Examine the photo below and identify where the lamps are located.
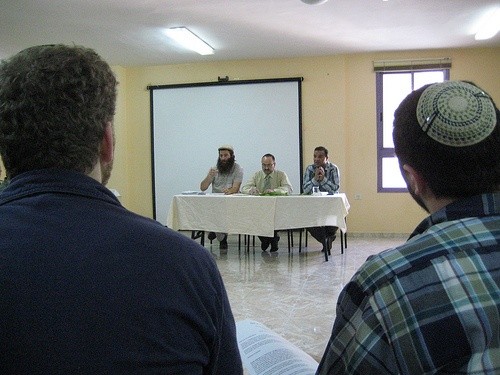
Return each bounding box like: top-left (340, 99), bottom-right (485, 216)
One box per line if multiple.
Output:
top-left (168, 24), bottom-right (215, 56)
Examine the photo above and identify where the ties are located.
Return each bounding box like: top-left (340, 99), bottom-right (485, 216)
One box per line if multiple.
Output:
top-left (264, 176), bottom-right (271, 189)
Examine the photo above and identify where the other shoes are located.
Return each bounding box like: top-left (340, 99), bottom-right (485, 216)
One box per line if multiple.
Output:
top-left (208, 232), bottom-right (217, 240)
top-left (261, 234), bottom-right (281, 252)
top-left (220, 235), bottom-right (228, 249)
top-left (321, 235), bottom-right (336, 252)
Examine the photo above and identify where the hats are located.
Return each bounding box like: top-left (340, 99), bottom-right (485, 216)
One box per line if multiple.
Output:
top-left (218, 144), bottom-right (234, 152)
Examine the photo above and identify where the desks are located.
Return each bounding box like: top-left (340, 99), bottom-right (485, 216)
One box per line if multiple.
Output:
top-left (165, 192), bottom-right (352, 258)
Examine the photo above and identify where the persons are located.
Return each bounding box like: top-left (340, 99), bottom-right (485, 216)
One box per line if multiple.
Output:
top-left (0, 42), bottom-right (244, 375)
top-left (302, 146), bottom-right (340, 251)
top-left (240, 153), bottom-right (292, 253)
top-left (317, 80), bottom-right (499, 375)
top-left (200, 146), bottom-right (244, 251)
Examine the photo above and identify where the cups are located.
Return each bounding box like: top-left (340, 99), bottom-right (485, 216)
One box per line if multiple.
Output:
top-left (251, 187), bottom-right (257, 196)
top-left (212, 166), bottom-right (218, 177)
top-left (313, 186), bottom-right (320, 196)
